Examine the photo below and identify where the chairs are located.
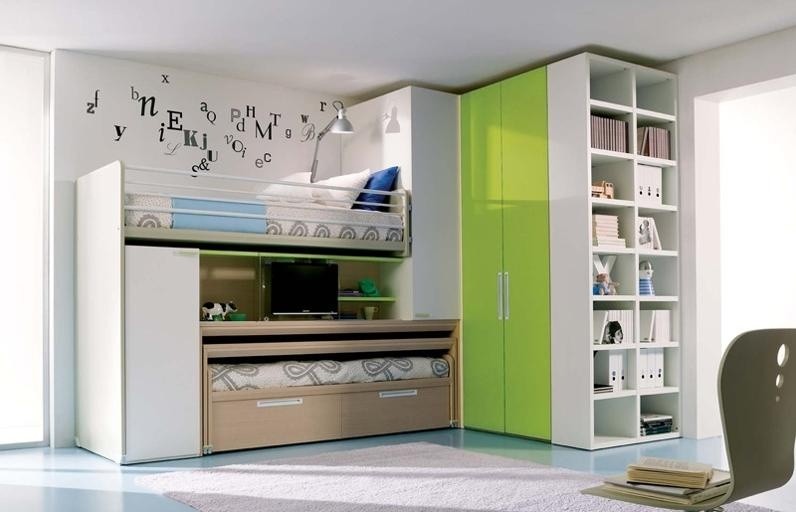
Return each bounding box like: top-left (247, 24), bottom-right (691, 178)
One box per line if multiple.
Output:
top-left (579, 328), bottom-right (795, 512)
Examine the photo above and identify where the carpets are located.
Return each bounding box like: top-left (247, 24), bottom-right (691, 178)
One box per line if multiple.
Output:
top-left (136, 440), bottom-right (777, 512)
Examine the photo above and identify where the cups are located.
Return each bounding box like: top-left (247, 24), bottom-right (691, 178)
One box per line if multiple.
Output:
top-left (364, 306), bottom-right (378, 320)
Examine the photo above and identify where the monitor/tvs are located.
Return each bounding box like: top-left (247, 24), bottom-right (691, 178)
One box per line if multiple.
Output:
top-left (271, 261), bottom-right (338, 319)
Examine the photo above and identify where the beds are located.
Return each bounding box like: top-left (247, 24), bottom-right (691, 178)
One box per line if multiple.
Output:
top-left (122, 159), bottom-right (411, 253)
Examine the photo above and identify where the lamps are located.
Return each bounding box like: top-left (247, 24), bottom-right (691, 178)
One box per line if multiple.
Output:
top-left (311, 108), bottom-right (354, 183)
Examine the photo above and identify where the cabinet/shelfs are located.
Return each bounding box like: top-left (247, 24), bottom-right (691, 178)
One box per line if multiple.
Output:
top-left (124, 245), bottom-right (460, 466)
top-left (460, 51), bottom-right (683, 450)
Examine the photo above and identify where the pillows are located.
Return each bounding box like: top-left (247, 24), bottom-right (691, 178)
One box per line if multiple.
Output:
top-left (254, 167), bottom-right (398, 211)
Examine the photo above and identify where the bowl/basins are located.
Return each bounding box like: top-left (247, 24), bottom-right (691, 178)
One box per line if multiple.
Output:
top-left (229, 313), bottom-right (248, 320)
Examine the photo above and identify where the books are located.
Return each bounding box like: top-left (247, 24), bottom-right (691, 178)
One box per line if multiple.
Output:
top-left (601, 484), bottom-right (732, 505)
top-left (601, 466), bottom-right (731, 495)
top-left (624, 455), bottom-right (713, 490)
top-left (589, 111), bottom-right (676, 439)
top-left (339, 289), bottom-right (361, 296)
